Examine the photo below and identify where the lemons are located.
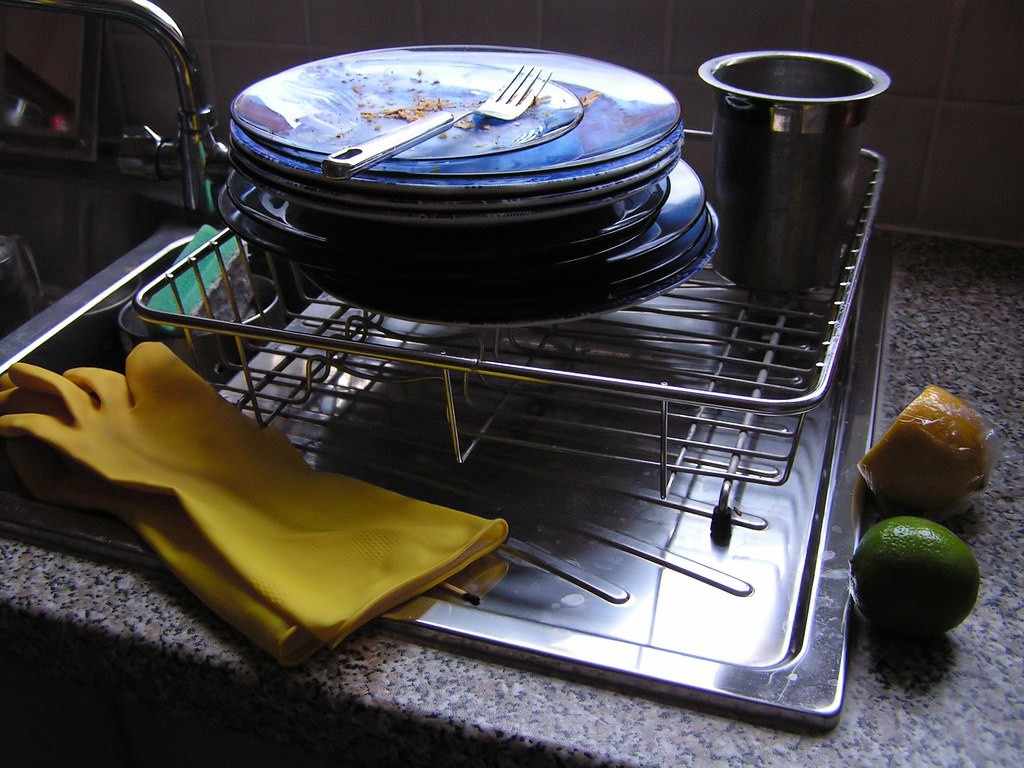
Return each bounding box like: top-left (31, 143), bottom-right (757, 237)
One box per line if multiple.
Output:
top-left (856, 385), bottom-right (993, 512)
top-left (849, 517), bottom-right (980, 634)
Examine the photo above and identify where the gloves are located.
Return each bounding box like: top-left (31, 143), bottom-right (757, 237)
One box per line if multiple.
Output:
top-left (0, 340), bottom-right (508, 667)
